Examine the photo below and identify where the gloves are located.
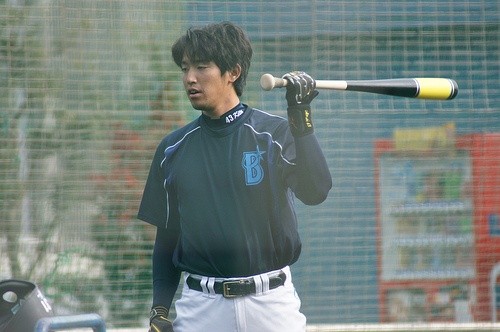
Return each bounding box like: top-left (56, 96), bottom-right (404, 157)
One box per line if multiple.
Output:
top-left (149, 306), bottom-right (173, 332)
top-left (282, 70), bottom-right (320, 139)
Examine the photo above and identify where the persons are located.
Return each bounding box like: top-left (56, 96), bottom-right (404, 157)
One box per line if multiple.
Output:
top-left (136, 20), bottom-right (333, 332)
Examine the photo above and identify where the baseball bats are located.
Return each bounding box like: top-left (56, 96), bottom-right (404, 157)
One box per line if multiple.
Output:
top-left (260, 73), bottom-right (459, 101)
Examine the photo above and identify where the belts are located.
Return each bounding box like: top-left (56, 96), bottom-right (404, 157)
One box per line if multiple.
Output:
top-left (185, 274), bottom-right (287, 298)
